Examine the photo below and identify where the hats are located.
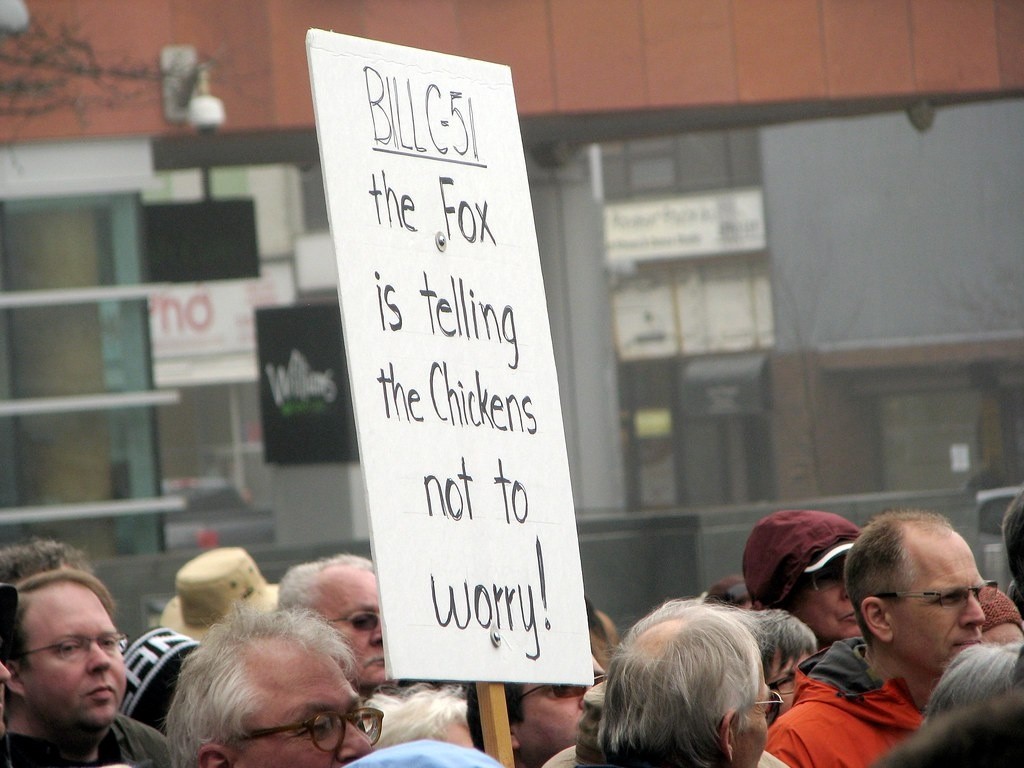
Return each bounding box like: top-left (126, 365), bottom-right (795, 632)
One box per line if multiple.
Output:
top-left (159, 544), bottom-right (282, 642)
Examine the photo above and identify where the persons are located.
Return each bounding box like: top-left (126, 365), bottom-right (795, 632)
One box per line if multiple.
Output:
top-left (0, 489), bottom-right (1024, 768)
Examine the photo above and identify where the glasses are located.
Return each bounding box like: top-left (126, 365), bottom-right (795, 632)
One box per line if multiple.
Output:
top-left (508, 672), bottom-right (609, 713)
top-left (753, 689), bottom-right (785, 714)
top-left (873, 579), bottom-right (1000, 612)
top-left (808, 569), bottom-right (843, 591)
top-left (766, 671), bottom-right (796, 698)
top-left (223, 705), bottom-right (384, 753)
top-left (331, 612), bottom-right (380, 632)
top-left (16, 631), bottom-right (130, 661)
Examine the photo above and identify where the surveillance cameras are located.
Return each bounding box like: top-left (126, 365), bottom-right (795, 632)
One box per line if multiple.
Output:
top-left (187, 96), bottom-right (225, 136)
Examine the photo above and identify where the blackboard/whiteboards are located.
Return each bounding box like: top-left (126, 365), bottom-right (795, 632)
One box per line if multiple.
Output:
top-left (304, 29), bottom-right (594, 684)
